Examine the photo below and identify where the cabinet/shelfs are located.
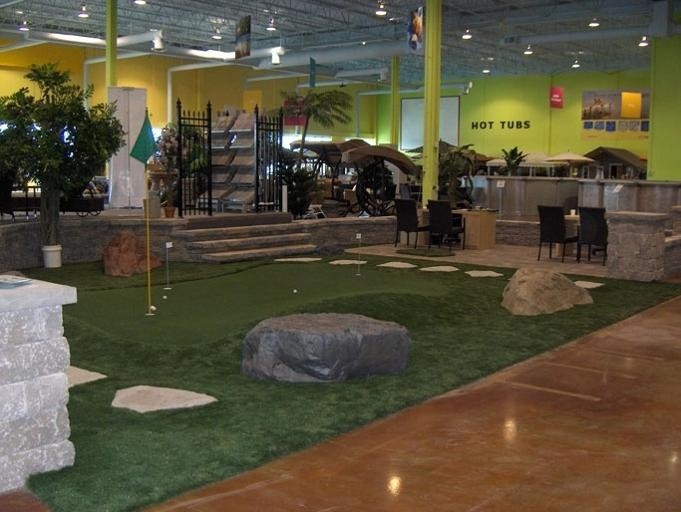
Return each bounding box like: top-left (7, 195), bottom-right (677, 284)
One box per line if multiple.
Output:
top-left (195, 113), bottom-right (255, 212)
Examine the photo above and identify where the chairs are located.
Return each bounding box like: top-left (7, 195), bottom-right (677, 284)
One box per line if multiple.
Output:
top-left (536, 205), bottom-right (606, 268)
top-left (392, 197), bottom-right (465, 251)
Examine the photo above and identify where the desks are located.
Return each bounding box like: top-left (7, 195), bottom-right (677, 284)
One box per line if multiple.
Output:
top-left (418, 207), bottom-right (497, 250)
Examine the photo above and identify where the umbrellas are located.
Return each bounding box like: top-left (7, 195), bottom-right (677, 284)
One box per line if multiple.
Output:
top-left (543, 149), bottom-right (596, 177)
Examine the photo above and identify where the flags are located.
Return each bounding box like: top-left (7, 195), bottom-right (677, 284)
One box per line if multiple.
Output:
top-left (130, 115), bottom-right (158, 165)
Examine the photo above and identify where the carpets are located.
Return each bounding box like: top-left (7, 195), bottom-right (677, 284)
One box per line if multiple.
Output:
top-left (21, 250), bottom-right (681, 512)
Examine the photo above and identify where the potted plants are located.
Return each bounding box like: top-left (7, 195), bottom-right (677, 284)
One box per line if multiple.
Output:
top-left (279, 89), bottom-right (354, 218)
top-left (0, 61), bottom-right (128, 268)
